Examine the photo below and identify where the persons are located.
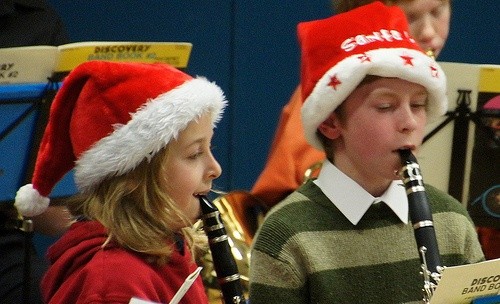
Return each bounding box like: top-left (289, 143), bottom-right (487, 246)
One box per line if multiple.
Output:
top-left (246, 1), bottom-right (488, 304)
top-left (252, 0), bottom-right (451, 210)
top-left (15, 61), bottom-right (222, 304)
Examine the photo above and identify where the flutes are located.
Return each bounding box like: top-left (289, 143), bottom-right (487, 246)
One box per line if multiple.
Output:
top-left (398, 149), bottom-right (447, 304)
top-left (199, 194), bottom-right (246, 304)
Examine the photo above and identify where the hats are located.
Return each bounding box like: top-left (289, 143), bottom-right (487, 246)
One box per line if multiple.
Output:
top-left (295, 4), bottom-right (448, 151)
top-left (12, 59), bottom-right (228, 221)
